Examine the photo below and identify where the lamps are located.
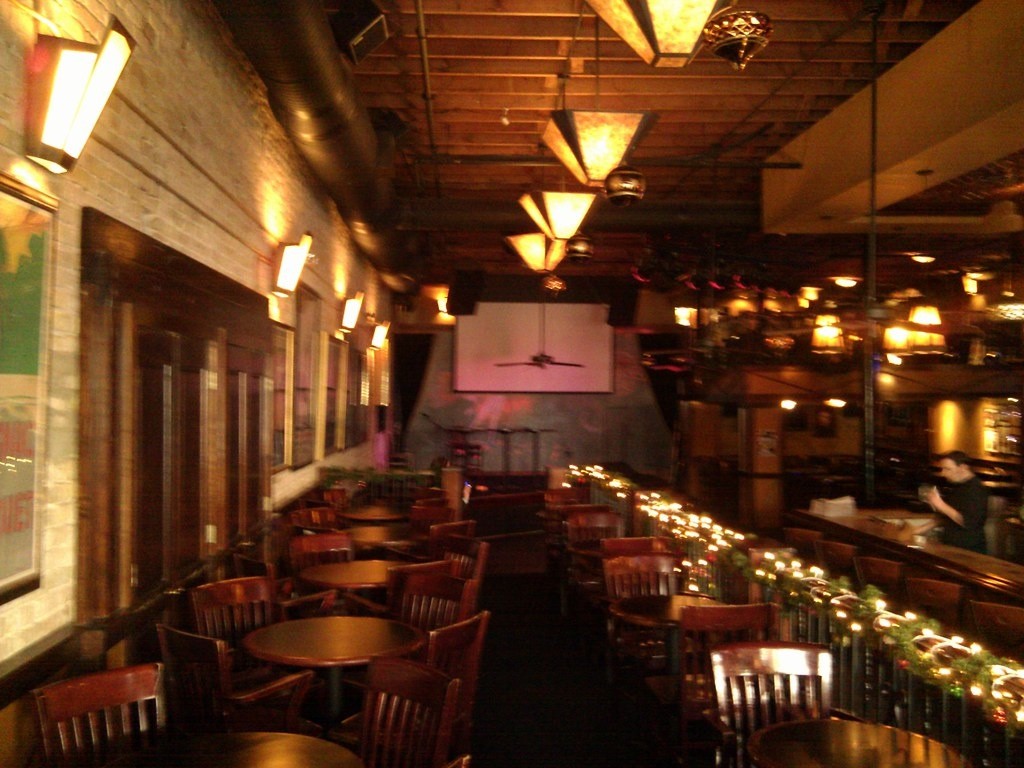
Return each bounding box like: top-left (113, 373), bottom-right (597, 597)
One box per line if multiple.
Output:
top-left (504, 233), bottom-right (567, 273)
top-left (632, 244), bottom-right (811, 297)
top-left (541, 15), bottom-right (661, 189)
top-left (24, 13), bottom-right (135, 174)
top-left (369, 320), bottom-right (391, 350)
top-left (517, 177), bottom-right (597, 241)
top-left (338, 290), bottom-right (365, 334)
top-left (583, 0), bottom-right (735, 68)
top-left (269, 234), bottom-right (313, 298)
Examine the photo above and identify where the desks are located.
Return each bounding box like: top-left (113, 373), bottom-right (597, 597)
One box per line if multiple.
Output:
top-left (341, 523), bottom-right (428, 559)
top-left (100, 730), bottom-right (368, 768)
top-left (296, 559), bottom-right (416, 615)
top-left (546, 503), bottom-right (607, 513)
top-left (336, 505), bottom-right (408, 523)
top-left (564, 538), bottom-right (601, 559)
top-left (608, 594), bottom-right (729, 734)
top-left (748, 719), bottom-right (967, 768)
top-left (239, 616), bottom-right (428, 724)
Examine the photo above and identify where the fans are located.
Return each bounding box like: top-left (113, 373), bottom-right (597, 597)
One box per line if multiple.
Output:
top-left (494, 291), bottom-right (587, 370)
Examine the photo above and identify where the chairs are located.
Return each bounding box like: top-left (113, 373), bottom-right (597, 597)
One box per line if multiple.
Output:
top-left (27, 458), bottom-right (1024, 768)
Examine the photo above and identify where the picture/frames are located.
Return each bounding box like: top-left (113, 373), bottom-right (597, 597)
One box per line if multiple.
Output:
top-left (0, 172), bottom-right (61, 607)
top-left (269, 318), bottom-right (391, 475)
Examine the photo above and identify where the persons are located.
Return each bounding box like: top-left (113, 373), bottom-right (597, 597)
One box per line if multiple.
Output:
top-left (918, 450), bottom-right (989, 554)
